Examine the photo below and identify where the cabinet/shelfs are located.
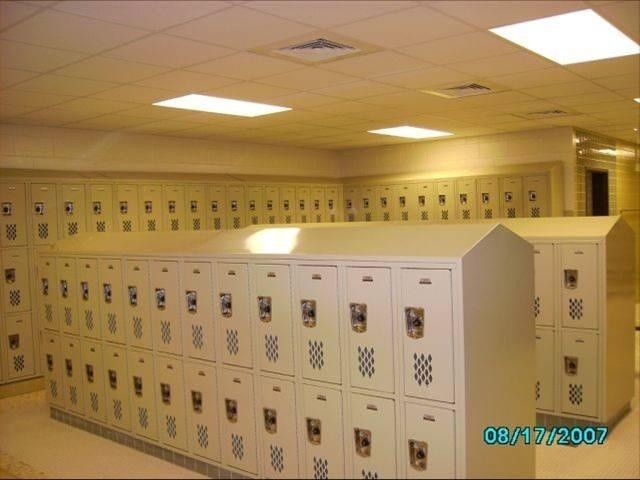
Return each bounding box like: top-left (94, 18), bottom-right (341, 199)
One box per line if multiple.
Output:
top-left (39, 225), bottom-right (536, 478)
top-left (343, 168), bottom-right (563, 224)
top-left (432, 214), bottom-right (638, 439)
top-left (0, 172), bottom-right (342, 396)
